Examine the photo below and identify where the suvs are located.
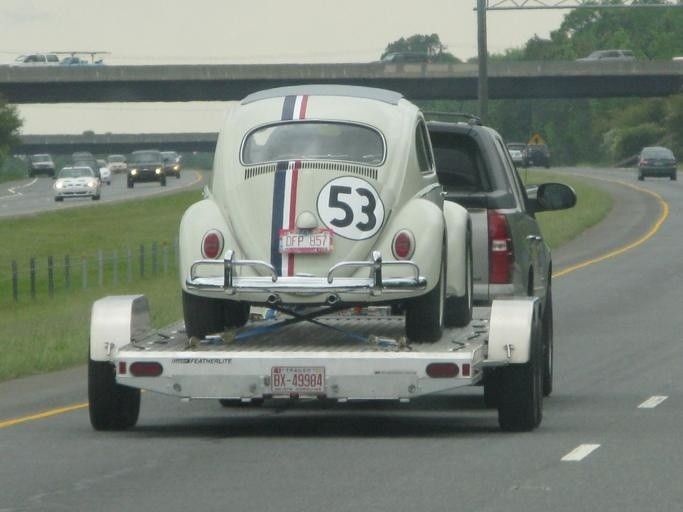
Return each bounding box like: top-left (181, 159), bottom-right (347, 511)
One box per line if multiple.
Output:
top-left (573, 46), bottom-right (640, 63)
top-left (504, 139), bottom-right (550, 167)
top-left (367, 49), bottom-right (433, 68)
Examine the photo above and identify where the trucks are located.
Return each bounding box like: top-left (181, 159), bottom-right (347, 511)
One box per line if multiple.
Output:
top-left (73, 105), bottom-right (577, 437)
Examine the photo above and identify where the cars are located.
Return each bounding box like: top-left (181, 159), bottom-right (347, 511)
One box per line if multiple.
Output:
top-left (635, 146), bottom-right (680, 184)
top-left (5, 47), bottom-right (118, 72)
top-left (26, 144), bottom-right (187, 208)
top-left (166, 81), bottom-right (479, 355)
